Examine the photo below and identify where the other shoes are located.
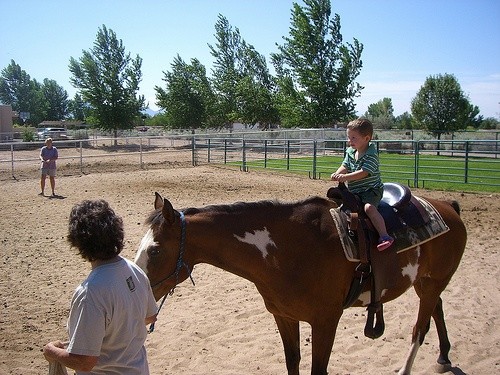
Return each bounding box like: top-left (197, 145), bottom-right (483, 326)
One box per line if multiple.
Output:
top-left (377, 236), bottom-right (394, 251)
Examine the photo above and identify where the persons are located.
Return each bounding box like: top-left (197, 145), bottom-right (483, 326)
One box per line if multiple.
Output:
top-left (331, 117), bottom-right (394, 252)
top-left (43, 199), bottom-right (159, 375)
top-left (38, 138), bottom-right (58, 198)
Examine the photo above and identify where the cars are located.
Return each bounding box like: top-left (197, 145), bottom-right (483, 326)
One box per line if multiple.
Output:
top-left (38, 127), bottom-right (67, 138)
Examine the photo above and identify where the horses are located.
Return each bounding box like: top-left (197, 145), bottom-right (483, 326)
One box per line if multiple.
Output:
top-left (132, 190), bottom-right (467, 375)
